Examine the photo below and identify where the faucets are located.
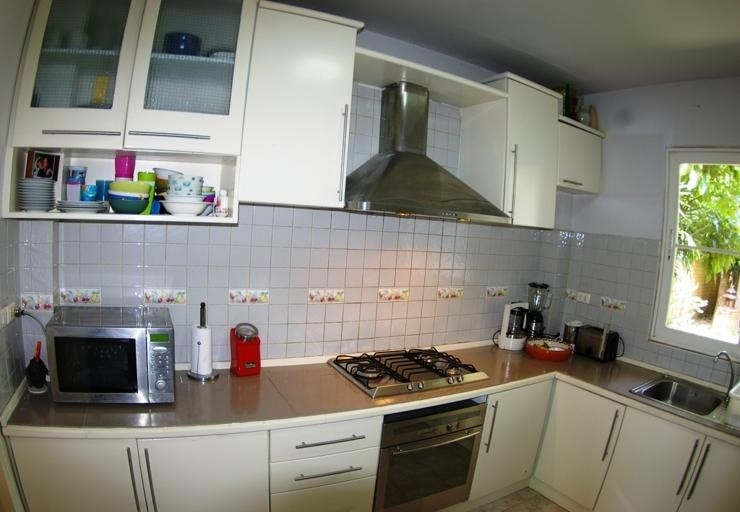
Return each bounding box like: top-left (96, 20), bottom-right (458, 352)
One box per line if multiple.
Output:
top-left (713, 350), bottom-right (735, 406)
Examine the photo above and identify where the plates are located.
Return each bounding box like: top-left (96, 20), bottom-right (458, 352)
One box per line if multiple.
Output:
top-left (17, 178), bottom-right (55, 213)
top-left (56, 200), bottom-right (107, 212)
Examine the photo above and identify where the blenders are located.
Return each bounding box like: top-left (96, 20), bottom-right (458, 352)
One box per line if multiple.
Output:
top-left (524, 281), bottom-right (552, 339)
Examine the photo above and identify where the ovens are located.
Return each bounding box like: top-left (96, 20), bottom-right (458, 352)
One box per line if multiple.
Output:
top-left (371, 402), bottom-right (487, 511)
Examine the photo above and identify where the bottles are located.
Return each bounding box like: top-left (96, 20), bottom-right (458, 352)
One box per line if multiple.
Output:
top-left (565, 83), bottom-right (573, 117)
top-left (26, 340), bottom-right (46, 389)
top-left (576, 99), bottom-right (590, 127)
top-left (588, 104), bottom-right (598, 129)
top-left (217, 190), bottom-right (230, 216)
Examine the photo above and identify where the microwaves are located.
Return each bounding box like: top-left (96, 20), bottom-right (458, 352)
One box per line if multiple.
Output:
top-left (45, 305), bottom-right (177, 405)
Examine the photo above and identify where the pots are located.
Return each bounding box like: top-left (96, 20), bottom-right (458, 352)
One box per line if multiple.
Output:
top-left (160, 31), bottom-right (202, 57)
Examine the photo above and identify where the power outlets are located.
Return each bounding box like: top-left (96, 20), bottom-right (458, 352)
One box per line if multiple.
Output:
top-left (0, 300), bottom-right (16, 331)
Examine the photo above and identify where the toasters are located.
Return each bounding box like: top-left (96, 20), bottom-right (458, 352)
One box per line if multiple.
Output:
top-left (574, 324), bottom-right (620, 362)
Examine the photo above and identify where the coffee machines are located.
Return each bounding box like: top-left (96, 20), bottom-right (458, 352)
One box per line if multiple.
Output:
top-left (498, 300), bottom-right (528, 351)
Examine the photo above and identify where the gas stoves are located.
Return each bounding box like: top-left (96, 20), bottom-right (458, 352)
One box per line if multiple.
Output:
top-left (326, 347), bottom-right (490, 400)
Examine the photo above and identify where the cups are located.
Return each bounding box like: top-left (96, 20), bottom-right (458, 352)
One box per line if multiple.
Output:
top-left (562, 323), bottom-right (583, 353)
top-left (82, 178), bottom-right (112, 215)
top-left (137, 171), bottom-right (158, 214)
top-left (112, 155), bottom-right (136, 181)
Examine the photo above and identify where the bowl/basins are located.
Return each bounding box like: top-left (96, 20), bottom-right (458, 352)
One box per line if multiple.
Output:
top-left (527, 337), bottom-right (571, 362)
top-left (109, 180), bottom-right (151, 215)
top-left (153, 167), bottom-right (216, 216)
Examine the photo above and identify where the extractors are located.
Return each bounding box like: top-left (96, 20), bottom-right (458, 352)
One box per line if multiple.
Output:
top-left (345, 78), bottom-right (513, 228)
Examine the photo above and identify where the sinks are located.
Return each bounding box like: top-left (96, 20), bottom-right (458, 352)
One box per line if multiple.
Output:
top-left (628, 374), bottom-right (723, 420)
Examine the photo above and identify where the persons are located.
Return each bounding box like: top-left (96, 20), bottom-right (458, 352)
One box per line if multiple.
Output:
top-left (34, 157), bottom-right (53, 178)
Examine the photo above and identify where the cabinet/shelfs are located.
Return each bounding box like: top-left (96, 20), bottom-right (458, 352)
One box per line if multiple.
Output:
top-left (458, 72), bottom-right (563, 231)
top-left (594, 407), bottom-right (739, 510)
top-left (270, 414), bottom-right (385, 511)
top-left (531, 381), bottom-right (626, 509)
top-left (1, 0), bottom-right (260, 225)
top-left (234, 0), bottom-right (368, 212)
top-left (558, 113), bottom-right (609, 198)
top-left (9, 430), bottom-right (270, 512)
top-left (468, 379), bottom-right (554, 504)
